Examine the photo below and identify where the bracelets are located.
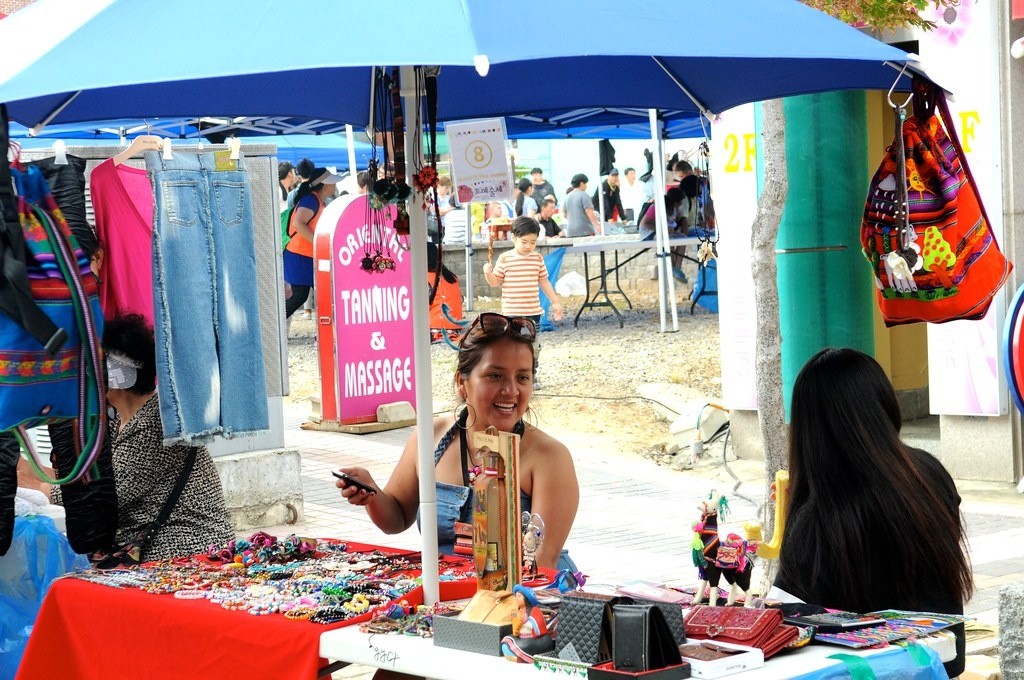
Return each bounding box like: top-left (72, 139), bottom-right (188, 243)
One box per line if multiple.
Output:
top-left (47, 531), bottom-right (448, 625)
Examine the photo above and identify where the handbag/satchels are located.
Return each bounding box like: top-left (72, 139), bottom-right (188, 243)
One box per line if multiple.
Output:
top-left (85, 541), bottom-right (144, 574)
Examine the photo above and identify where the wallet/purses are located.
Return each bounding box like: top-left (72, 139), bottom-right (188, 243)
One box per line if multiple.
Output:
top-left (679, 643), bottom-right (748, 662)
top-left (612, 603), bottom-right (682, 673)
top-left (684, 603), bottom-right (799, 660)
top-left (633, 598), bottom-right (686, 644)
top-left (554, 597), bottom-right (613, 666)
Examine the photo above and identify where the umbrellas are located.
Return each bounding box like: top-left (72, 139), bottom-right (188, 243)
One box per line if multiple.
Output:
top-left (0, 0), bottom-right (959, 615)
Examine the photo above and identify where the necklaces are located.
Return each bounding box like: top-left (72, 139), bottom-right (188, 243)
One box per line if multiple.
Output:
top-left (466, 440), bottom-right (482, 484)
top-left (608, 182), bottom-right (616, 191)
top-left (359, 67), bottom-right (439, 273)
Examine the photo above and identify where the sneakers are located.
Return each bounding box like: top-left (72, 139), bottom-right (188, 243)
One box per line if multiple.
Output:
top-left (674, 268), bottom-right (687, 283)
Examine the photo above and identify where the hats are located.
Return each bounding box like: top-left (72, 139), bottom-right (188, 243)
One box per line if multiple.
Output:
top-left (610, 168), bottom-right (619, 174)
top-left (278, 162), bottom-right (296, 174)
top-left (309, 170), bottom-right (344, 188)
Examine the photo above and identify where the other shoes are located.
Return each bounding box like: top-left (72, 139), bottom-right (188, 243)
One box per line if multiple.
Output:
top-left (650, 265), bottom-right (658, 279)
top-left (532, 381), bottom-right (544, 390)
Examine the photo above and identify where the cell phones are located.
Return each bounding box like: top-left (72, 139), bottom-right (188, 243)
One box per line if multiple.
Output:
top-left (331, 469), bottom-right (378, 495)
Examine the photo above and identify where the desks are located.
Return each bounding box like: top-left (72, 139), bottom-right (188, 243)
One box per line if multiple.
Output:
top-left (14, 538), bottom-right (956, 680)
top-left (563, 233), bottom-right (718, 328)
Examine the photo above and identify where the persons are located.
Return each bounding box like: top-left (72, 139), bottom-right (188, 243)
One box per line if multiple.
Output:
top-left (427, 177), bottom-right (467, 243)
top-left (562, 174), bottom-right (601, 236)
top-left (278, 158), bottom-right (395, 337)
top-left (483, 215), bottom-right (561, 389)
top-left (471, 167), bottom-right (564, 238)
top-left (427, 242), bottom-right (463, 341)
top-left (335, 313), bottom-right (580, 680)
top-left (17, 315), bottom-right (234, 562)
top-left (773, 348), bottom-right (973, 678)
top-left (592, 151), bottom-right (714, 283)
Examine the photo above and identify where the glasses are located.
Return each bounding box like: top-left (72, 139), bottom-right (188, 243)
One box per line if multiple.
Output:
top-left (458, 313), bottom-right (537, 351)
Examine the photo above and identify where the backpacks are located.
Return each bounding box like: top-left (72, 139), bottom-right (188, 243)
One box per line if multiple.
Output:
top-left (0, 142), bottom-right (111, 486)
top-left (861, 76), bottom-right (1013, 328)
top-left (280, 194), bottom-right (320, 253)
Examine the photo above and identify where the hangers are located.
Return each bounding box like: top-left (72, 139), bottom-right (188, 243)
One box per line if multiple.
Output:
top-left (162, 116), bottom-right (240, 160)
top-left (113, 118), bottom-right (173, 166)
top-left (8, 137), bottom-right (71, 164)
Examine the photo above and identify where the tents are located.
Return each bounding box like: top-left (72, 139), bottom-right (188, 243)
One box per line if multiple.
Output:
top-left (7, 108), bottom-right (713, 333)
